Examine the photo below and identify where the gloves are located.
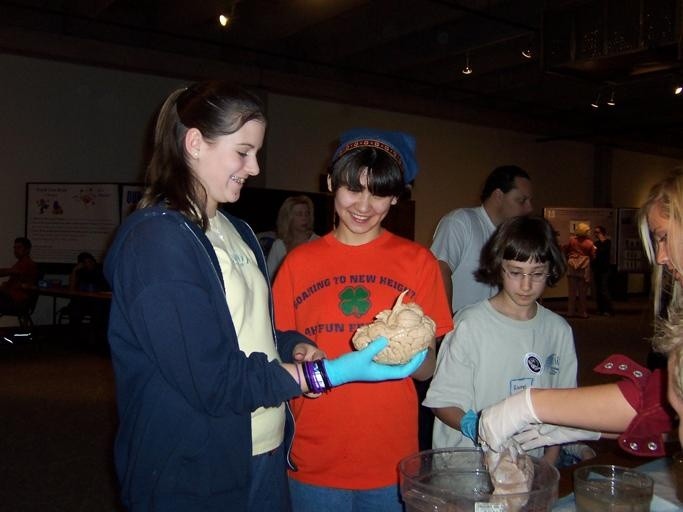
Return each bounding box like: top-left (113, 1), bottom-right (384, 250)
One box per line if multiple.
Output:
top-left (323, 335), bottom-right (430, 388)
top-left (461, 388), bottom-right (602, 458)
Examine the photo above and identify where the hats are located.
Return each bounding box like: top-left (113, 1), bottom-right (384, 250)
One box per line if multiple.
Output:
top-left (331, 127), bottom-right (417, 182)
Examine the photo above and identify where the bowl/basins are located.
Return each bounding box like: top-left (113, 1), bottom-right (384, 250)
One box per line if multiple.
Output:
top-left (392, 446), bottom-right (561, 510)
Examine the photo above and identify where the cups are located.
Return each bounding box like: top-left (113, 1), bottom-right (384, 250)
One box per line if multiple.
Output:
top-left (572, 463), bottom-right (655, 511)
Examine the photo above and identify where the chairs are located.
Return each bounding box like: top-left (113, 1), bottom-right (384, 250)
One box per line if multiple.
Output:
top-left (1, 275), bottom-right (42, 351)
top-left (58, 294), bottom-right (94, 345)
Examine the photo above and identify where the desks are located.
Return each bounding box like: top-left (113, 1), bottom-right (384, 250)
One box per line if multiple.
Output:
top-left (519, 436), bottom-right (682, 512)
top-left (20, 283), bottom-right (114, 344)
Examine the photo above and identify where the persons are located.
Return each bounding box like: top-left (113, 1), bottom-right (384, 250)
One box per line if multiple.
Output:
top-left (590, 224), bottom-right (613, 317)
top-left (413, 159), bottom-right (533, 475)
top-left (65, 252), bottom-right (114, 333)
top-left (271, 120), bottom-right (459, 510)
top-left (653, 308), bottom-right (682, 469)
top-left (96, 76), bottom-right (432, 509)
top-left (464, 161), bottom-right (683, 458)
top-left (422, 206), bottom-right (582, 510)
top-left (258, 191), bottom-right (325, 284)
top-left (0, 231), bottom-right (41, 320)
top-left (562, 218), bottom-right (597, 319)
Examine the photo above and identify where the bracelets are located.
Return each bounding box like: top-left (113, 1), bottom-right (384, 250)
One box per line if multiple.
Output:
top-left (291, 358), bottom-right (332, 394)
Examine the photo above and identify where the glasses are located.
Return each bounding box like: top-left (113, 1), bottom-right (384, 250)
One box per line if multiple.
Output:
top-left (499, 261), bottom-right (552, 284)
